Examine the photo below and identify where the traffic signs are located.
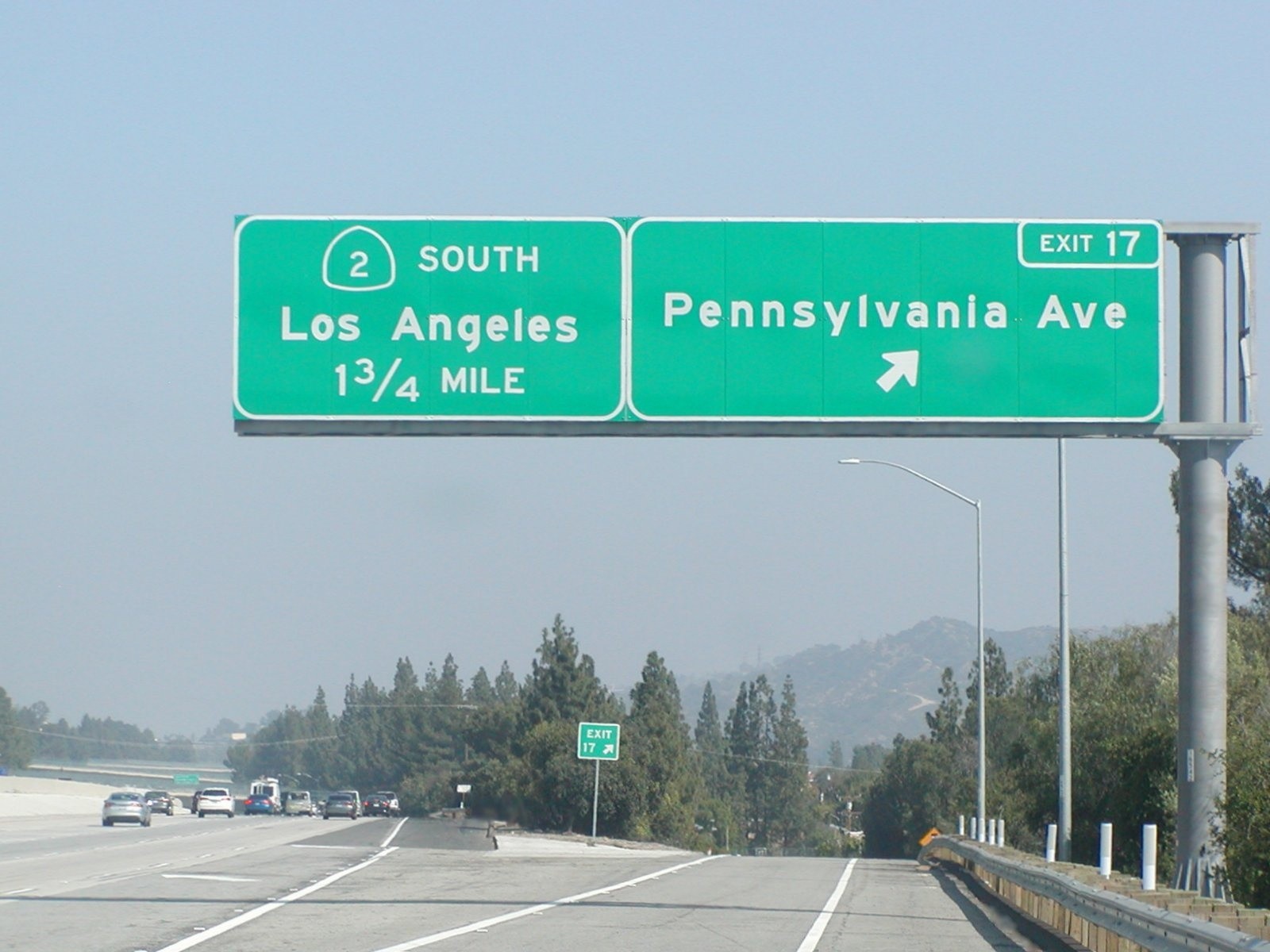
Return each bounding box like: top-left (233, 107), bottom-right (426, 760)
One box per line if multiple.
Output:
top-left (232, 217), bottom-right (1164, 428)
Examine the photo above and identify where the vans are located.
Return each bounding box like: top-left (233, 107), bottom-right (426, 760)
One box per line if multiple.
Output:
top-left (338, 790), bottom-right (362, 816)
top-left (251, 780), bottom-right (281, 813)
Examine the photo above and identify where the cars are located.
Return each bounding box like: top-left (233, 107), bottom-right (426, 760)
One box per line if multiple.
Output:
top-left (144, 790), bottom-right (174, 816)
top-left (197, 786), bottom-right (234, 817)
top-left (324, 793), bottom-right (358, 819)
top-left (377, 789), bottom-right (400, 815)
top-left (285, 791), bottom-right (310, 815)
top-left (191, 790), bottom-right (201, 814)
top-left (103, 791), bottom-right (152, 826)
top-left (365, 795), bottom-right (391, 816)
top-left (243, 794), bottom-right (275, 816)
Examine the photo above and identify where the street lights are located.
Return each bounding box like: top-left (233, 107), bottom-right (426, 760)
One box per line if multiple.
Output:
top-left (838, 456), bottom-right (988, 843)
